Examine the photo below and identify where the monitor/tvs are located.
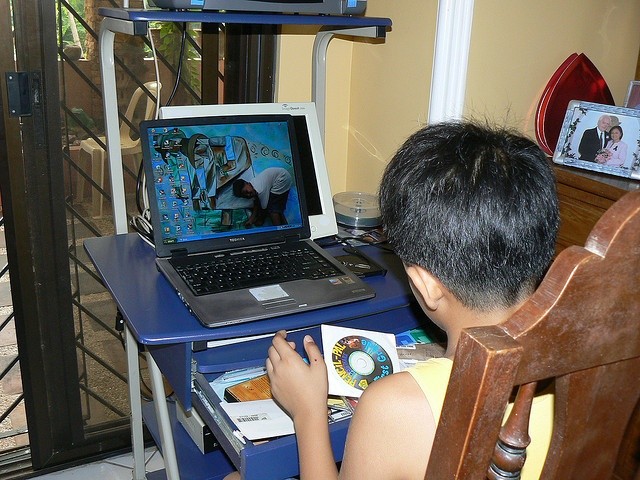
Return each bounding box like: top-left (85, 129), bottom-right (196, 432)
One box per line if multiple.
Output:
top-left (145, 0), bottom-right (369, 14)
top-left (158, 101), bottom-right (340, 240)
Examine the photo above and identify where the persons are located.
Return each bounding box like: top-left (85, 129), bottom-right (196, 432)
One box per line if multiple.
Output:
top-left (600, 126), bottom-right (627, 168)
top-left (266, 100), bottom-right (557, 476)
top-left (234, 168), bottom-right (292, 225)
top-left (578, 117), bottom-right (609, 161)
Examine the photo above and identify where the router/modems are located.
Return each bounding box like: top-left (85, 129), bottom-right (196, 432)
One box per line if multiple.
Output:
top-left (175, 399), bottom-right (221, 456)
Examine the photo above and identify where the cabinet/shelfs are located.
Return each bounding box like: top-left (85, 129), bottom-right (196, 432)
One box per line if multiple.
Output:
top-left (543, 155), bottom-right (640, 275)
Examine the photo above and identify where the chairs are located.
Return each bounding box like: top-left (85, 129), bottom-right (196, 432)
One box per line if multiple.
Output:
top-left (424, 187), bottom-right (640, 479)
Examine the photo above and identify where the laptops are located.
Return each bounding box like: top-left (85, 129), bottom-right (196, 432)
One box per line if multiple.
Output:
top-left (138, 114), bottom-right (388, 330)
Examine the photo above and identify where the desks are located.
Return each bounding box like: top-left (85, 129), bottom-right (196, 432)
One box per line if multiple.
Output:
top-left (81, 6), bottom-right (448, 479)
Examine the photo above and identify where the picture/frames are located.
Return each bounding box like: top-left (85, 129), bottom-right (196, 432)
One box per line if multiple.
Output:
top-left (552, 99), bottom-right (640, 179)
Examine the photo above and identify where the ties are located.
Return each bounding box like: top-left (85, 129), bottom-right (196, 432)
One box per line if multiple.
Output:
top-left (600, 133), bottom-right (603, 149)
